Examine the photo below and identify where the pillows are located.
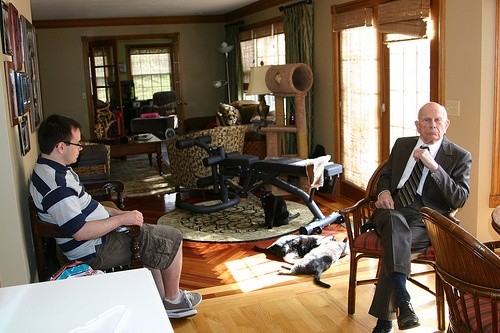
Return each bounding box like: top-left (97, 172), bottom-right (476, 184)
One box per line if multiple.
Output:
top-left (215, 102), bottom-right (242, 126)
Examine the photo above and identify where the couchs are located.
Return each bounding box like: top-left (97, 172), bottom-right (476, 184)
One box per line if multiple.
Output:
top-left (131, 90), bottom-right (176, 137)
top-left (169, 99), bottom-right (276, 190)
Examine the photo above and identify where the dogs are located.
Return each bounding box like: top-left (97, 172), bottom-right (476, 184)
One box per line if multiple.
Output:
top-left (253, 235), bottom-right (327, 265)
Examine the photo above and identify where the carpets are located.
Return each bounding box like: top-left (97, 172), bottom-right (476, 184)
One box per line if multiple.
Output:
top-left (157, 199), bottom-right (315, 243)
top-left (111, 157), bottom-right (178, 198)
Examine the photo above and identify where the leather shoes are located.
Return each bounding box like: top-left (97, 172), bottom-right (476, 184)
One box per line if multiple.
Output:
top-left (395, 301), bottom-right (420, 330)
top-left (372, 319), bottom-right (392, 333)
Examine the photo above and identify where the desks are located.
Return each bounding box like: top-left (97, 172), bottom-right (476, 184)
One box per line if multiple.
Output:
top-left (0, 267), bottom-right (175, 333)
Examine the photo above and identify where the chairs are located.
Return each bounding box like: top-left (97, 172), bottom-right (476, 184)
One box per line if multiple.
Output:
top-left (420, 207), bottom-right (500, 333)
top-left (338, 158), bottom-right (460, 331)
top-left (27, 137), bottom-right (142, 280)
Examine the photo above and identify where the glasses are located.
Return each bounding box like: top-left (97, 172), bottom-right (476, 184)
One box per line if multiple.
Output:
top-left (56, 140), bottom-right (85, 151)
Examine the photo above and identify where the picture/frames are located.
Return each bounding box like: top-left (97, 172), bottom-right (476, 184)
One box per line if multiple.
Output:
top-left (0, 0), bottom-right (43, 155)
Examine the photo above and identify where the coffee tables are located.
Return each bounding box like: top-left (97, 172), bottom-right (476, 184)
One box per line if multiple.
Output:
top-left (91, 132), bottom-right (163, 176)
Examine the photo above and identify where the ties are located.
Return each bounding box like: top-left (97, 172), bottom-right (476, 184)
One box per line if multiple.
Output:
top-left (397, 146), bottom-right (429, 207)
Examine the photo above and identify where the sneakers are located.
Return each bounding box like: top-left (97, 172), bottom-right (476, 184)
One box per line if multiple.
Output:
top-left (180, 290), bottom-right (201, 307)
top-left (163, 292), bottom-right (197, 318)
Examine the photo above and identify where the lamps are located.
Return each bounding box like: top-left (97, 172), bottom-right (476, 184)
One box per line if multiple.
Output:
top-left (213, 42), bottom-right (234, 104)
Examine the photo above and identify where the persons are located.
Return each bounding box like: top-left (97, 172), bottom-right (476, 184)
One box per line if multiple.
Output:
top-left (30, 115), bottom-right (203, 319)
top-left (360, 102), bottom-right (472, 333)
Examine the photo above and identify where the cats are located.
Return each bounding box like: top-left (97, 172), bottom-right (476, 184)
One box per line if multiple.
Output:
top-left (276, 234), bottom-right (349, 288)
